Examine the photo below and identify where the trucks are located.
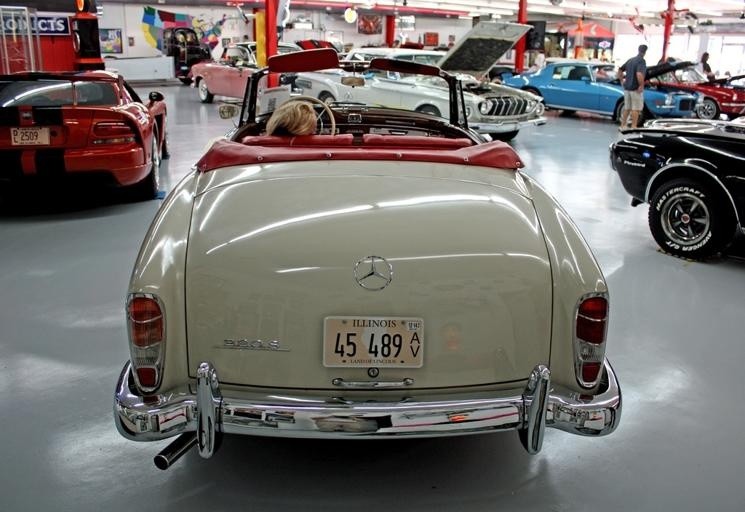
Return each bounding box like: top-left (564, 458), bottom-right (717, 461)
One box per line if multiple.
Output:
top-left (0, 0), bottom-right (107, 75)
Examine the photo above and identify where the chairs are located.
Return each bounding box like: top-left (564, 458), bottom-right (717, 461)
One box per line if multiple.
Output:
top-left (241, 133), bottom-right (354, 147)
top-left (361, 133), bottom-right (473, 150)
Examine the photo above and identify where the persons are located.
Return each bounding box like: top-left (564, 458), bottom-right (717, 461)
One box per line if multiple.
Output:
top-left (618, 45), bottom-right (648, 128)
top-left (266, 100), bottom-right (317, 137)
top-left (700, 52), bottom-right (715, 82)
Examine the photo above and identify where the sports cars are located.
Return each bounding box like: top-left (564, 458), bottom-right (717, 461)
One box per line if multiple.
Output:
top-left (94, 37), bottom-right (630, 494)
top-left (1, 60), bottom-right (164, 206)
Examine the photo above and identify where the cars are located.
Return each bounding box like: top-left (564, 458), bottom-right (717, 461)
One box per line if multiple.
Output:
top-left (164, 23), bottom-right (213, 86)
